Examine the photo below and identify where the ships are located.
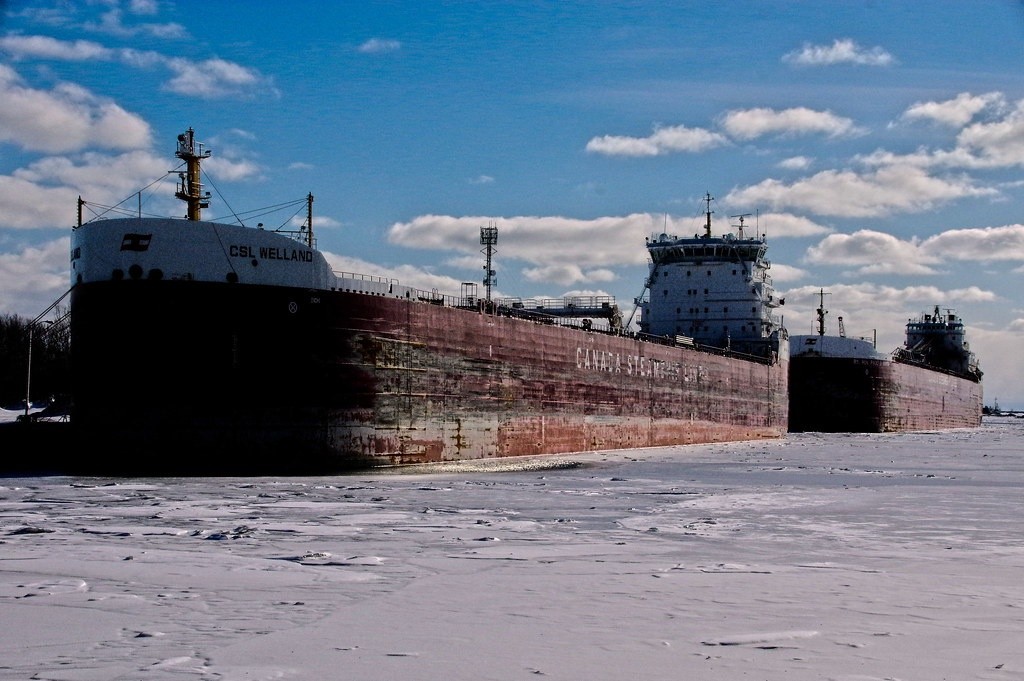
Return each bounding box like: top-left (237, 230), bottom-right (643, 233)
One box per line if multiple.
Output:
top-left (789, 285), bottom-right (986, 433)
top-left (64, 126), bottom-right (792, 473)
